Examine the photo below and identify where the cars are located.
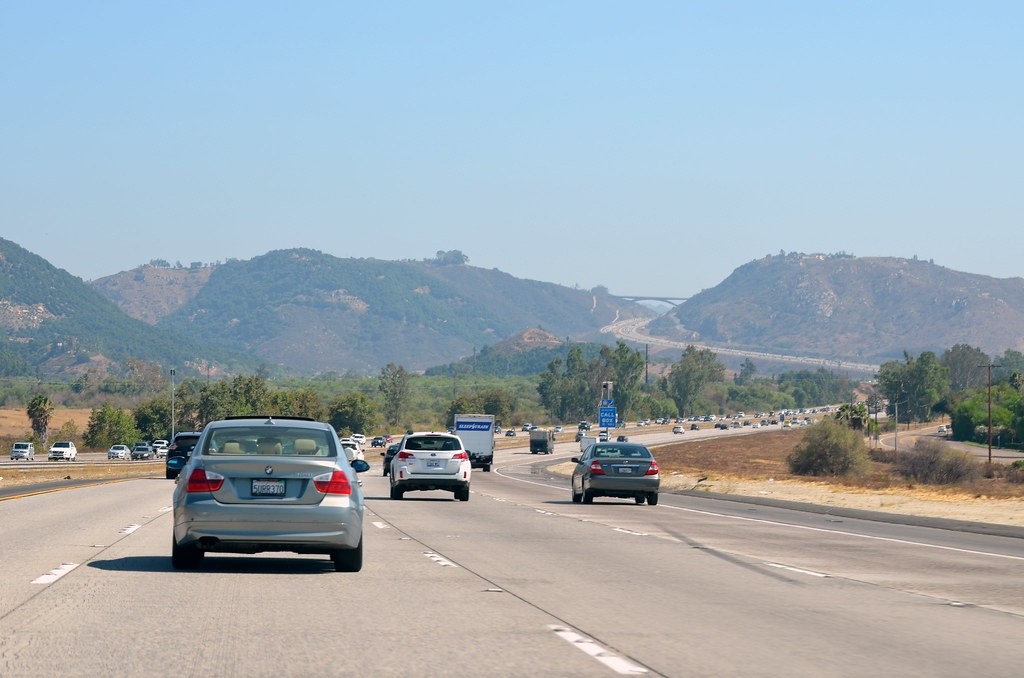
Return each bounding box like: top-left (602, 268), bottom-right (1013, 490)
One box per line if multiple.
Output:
top-left (380, 444), bottom-right (400, 476)
top-left (172, 416), bottom-right (370, 572)
top-left (371, 435), bottom-right (393, 448)
top-left (165, 432), bottom-right (218, 479)
top-left (108, 445), bottom-right (131, 460)
top-left (10, 442), bottom-right (34, 461)
top-left (495, 426), bottom-right (501, 434)
top-left (48, 442), bottom-right (77, 461)
top-left (132, 446), bottom-right (155, 460)
top-left (571, 443), bottom-right (660, 505)
top-left (522, 407), bottom-right (833, 443)
top-left (505, 431), bottom-right (516, 436)
top-left (341, 433), bottom-right (366, 445)
top-left (131, 440), bottom-right (169, 458)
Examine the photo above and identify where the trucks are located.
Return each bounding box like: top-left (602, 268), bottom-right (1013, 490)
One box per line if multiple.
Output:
top-left (454, 414), bottom-right (496, 472)
top-left (529, 428), bottom-right (556, 454)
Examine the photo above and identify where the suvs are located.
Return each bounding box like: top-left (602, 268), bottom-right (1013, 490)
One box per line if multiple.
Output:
top-left (387, 430), bottom-right (471, 501)
top-left (341, 441), bottom-right (365, 463)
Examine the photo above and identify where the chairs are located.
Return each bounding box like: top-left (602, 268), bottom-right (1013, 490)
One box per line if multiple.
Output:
top-left (442, 442), bottom-right (455, 450)
top-left (257, 443), bottom-right (282, 454)
top-left (293, 438), bottom-right (317, 456)
top-left (411, 443), bottom-right (422, 450)
top-left (224, 443), bottom-right (249, 454)
